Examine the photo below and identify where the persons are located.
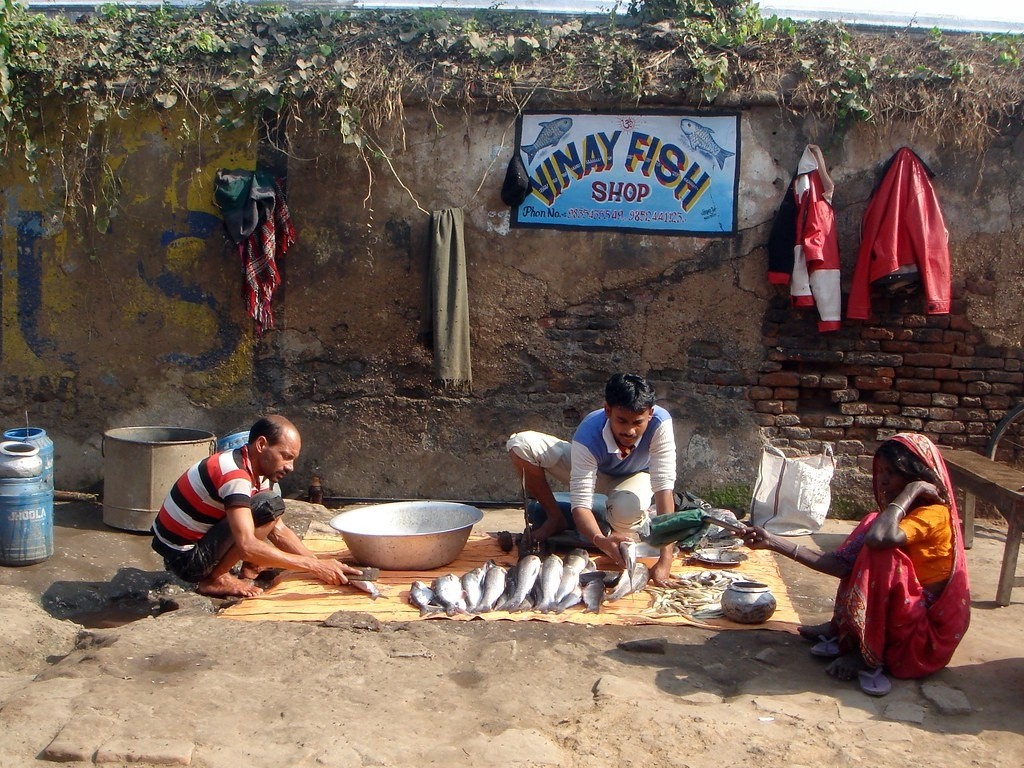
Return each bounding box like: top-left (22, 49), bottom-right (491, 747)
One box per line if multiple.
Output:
top-left (149, 414), bottom-right (366, 600)
top-left (731, 434), bottom-right (973, 682)
top-left (506, 371), bottom-right (691, 590)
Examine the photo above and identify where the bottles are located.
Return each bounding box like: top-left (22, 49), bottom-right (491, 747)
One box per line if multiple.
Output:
top-left (0, 442), bottom-right (44, 480)
top-left (721, 581), bottom-right (777, 624)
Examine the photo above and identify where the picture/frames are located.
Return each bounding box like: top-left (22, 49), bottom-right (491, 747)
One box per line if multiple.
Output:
top-left (510, 110), bottom-right (742, 239)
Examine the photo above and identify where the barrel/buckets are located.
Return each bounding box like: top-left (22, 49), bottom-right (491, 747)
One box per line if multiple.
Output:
top-left (217, 431), bottom-right (283, 500)
top-left (2, 427), bottom-right (54, 486)
top-left (0, 475), bottom-right (55, 566)
top-left (101, 426), bottom-right (218, 533)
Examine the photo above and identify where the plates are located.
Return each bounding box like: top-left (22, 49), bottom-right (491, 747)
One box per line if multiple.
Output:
top-left (695, 548), bottom-right (748, 565)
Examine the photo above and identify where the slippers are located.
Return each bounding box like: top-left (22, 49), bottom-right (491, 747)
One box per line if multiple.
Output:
top-left (809, 633), bottom-right (841, 658)
top-left (858, 667), bottom-right (893, 697)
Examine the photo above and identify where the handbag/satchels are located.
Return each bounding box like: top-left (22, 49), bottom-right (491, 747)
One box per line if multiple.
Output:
top-left (748, 441), bottom-right (835, 537)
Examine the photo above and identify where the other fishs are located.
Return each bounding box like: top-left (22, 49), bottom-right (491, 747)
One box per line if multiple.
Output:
top-left (350, 580), bottom-right (389, 601)
top-left (406, 538), bottom-right (756, 625)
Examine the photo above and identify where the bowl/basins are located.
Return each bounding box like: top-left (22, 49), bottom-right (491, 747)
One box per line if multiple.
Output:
top-left (329, 500), bottom-right (484, 571)
top-left (631, 540), bottom-right (680, 570)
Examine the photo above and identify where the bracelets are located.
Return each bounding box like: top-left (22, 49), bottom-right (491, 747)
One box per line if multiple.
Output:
top-left (794, 543), bottom-right (800, 561)
top-left (888, 503), bottom-right (906, 517)
top-left (593, 534), bottom-right (603, 546)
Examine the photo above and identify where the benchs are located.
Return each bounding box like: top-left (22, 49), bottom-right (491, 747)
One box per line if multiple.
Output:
top-left (937, 450), bottom-right (1024, 605)
